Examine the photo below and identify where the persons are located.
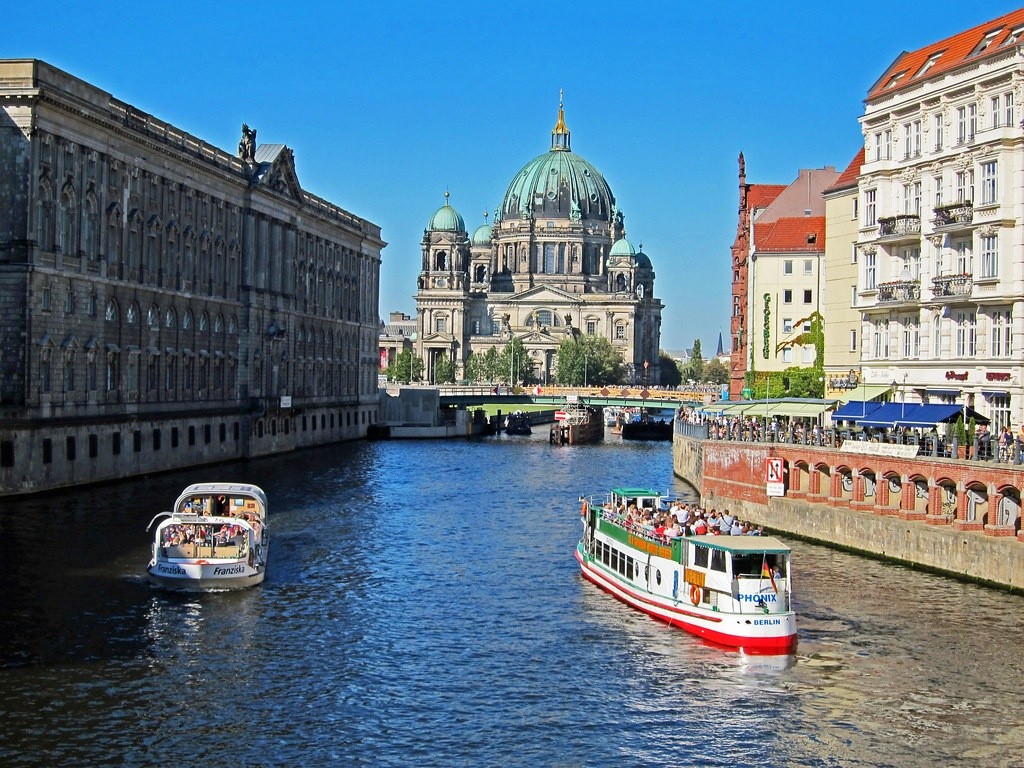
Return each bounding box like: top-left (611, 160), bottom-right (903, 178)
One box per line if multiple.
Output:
top-left (504, 407), bottom-right (528, 428)
top-left (548, 379), bottom-right (726, 394)
top-left (610, 408), bottom-right (1014, 458)
top-left (236, 123), bottom-right (259, 164)
top-left (161, 494), bottom-right (263, 548)
top-left (1017, 424), bottom-right (1024, 464)
top-left (579, 496), bottom-right (787, 611)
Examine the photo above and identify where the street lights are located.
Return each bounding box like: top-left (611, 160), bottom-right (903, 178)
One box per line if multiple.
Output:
top-left (760, 371), bottom-right (978, 459)
top-left (405, 344), bottom-right (591, 387)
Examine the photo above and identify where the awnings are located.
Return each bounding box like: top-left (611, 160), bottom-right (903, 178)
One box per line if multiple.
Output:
top-left (894, 401), bottom-right (993, 437)
top-left (855, 400), bottom-right (926, 443)
top-left (701, 400), bottom-right (845, 425)
top-left (830, 400), bottom-right (884, 427)
top-left (832, 381), bottom-right (894, 404)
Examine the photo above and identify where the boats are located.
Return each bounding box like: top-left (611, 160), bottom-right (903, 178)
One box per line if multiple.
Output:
top-left (144, 481), bottom-right (272, 592)
top-left (575, 485), bottom-right (800, 649)
top-left (502, 403), bottom-right (674, 441)
